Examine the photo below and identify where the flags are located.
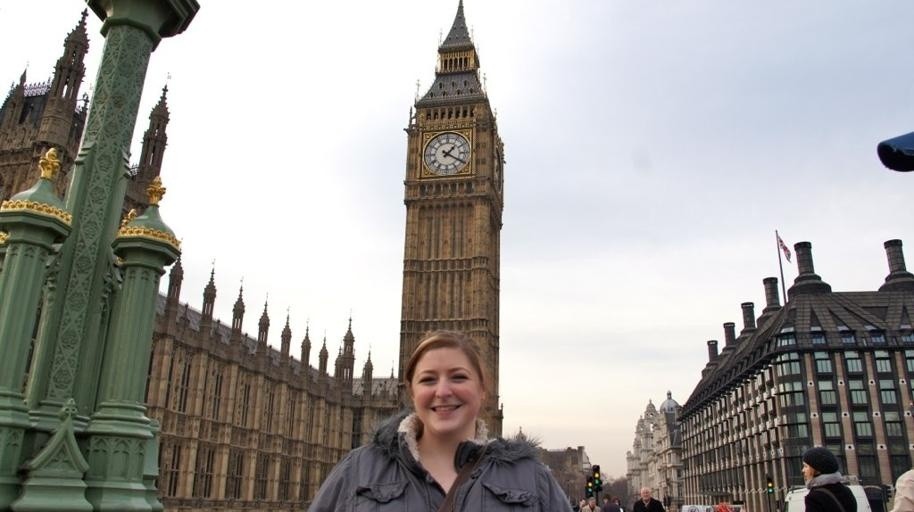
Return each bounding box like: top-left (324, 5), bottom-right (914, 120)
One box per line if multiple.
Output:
top-left (777, 235), bottom-right (791, 263)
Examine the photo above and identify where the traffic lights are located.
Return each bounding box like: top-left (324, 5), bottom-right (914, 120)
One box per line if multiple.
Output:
top-left (767, 477), bottom-right (774, 494)
top-left (585, 464), bottom-right (603, 499)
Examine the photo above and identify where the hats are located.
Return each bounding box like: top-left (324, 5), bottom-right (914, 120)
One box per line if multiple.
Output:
top-left (803, 447), bottom-right (839, 473)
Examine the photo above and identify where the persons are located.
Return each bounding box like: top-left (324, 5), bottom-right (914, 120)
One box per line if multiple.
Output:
top-left (800, 447), bottom-right (857, 512)
top-left (572, 486), bottom-right (672, 512)
top-left (889, 468), bottom-right (914, 512)
top-left (306, 328), bottom-right (574, 512)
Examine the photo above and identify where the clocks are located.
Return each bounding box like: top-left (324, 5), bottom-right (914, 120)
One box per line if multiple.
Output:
top-left (422, 131), bottom-right (469, 177)
